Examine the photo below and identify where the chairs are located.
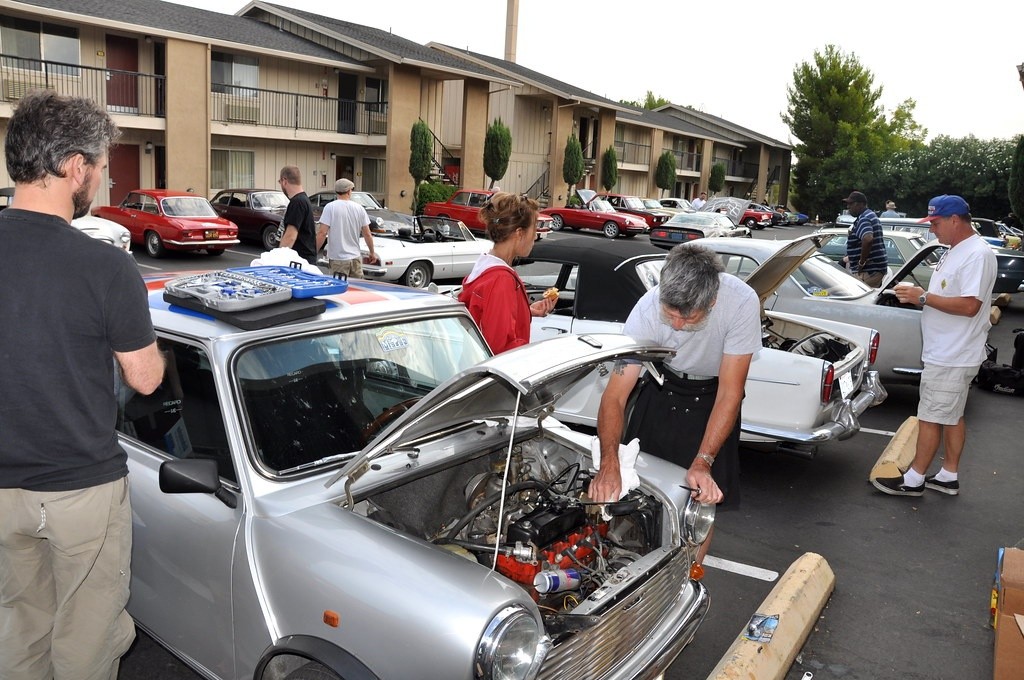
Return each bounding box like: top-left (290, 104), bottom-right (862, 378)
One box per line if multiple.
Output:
top-left (395, 228), bottom-right (414, 240)
top-left (422, 234), bottom-right (437, 243)
top-left (572, 204), bottom-right (579, 209)
top-left (173, 369), bottom-right (235, 482)
top-left (470, 197), bottom-right (479, 206)
top-left (581, 204), bottom-right (587, 209)
top-left (221, 195), bottom-right (241, 207)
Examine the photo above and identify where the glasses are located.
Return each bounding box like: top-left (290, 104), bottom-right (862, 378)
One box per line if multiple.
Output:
top-left (278, 177), bottom-right (286, 183)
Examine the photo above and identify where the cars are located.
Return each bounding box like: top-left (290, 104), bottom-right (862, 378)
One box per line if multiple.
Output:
top-left (207, 187), bottom-right (325, 251)
top-left (90, 188), bottom-right (242, 260)
top-left (0, 187), bottom-right (133, 256)
top-left (664, 196), bottom-right (1024, 294)
top-left (525, 235), bottom-right (952, 385)
top-left (423, 189), bottom-right (555, 242)
top-left (114, 267), bottom-right (717, 680)
top-left (650, 195), bottom-right (752, 246)
top-left (437, 237), bottom-right (890, 460)
top-left (307, 190), bottom-right (422, 236)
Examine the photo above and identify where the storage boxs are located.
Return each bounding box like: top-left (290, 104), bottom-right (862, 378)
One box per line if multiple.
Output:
top-left (993, 547), bottom-right (1024, 680)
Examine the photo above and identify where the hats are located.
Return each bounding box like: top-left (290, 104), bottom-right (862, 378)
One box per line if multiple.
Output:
top-left (887, 202), bottom-right (896, 209)
top-left (843, 192), bottom-right (867, 203)
top-left (918, 194), bottom-right (970, 222)
top-left (335, 178), bottom-right (354, 192)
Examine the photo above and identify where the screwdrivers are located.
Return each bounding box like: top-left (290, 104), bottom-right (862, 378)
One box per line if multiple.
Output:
top-left (679, 484), bottom-right (702, 493)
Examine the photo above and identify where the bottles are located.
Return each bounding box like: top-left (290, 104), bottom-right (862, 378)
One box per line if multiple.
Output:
top-left (534, 567), bottom-right (581, 593)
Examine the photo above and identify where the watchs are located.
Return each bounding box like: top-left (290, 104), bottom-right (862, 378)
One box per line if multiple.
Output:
top-left (696, 453), bottom-right (714, 466)
top-left (919, 292), bottom-right (929, 304)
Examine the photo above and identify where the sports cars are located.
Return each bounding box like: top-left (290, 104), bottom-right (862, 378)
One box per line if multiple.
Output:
top-left (563, 189), bottom-right (673, 235)
top-left (538, 199), bottom-right (650, 240)
top-left (316, 214), bottom-right (495, 289)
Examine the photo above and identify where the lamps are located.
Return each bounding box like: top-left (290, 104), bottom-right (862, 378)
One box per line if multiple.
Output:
top-left (187, 188), bottom-right (194, 193)
top-left (145, 35), bottom-right (152, 44)
top-left (330, 152), bottom-right (337, 161)
top-left (692, 180), bottom-right (695, 186)
top-left (145, 140), bottom-right (153, 155)
top-left (590, 115), bottom-right (595, 120)
top-left (334, 67), bottom-right (339, 74)
top-left (542, 106), bottom-right (548, 112)
top-left (401, 189), bottom-right (406, 198)
top-left (559, 195), bottom-right (563, 201)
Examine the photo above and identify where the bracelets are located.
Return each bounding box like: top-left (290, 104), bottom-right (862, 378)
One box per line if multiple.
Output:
top-left (858, 260), bottom-right (866, 266)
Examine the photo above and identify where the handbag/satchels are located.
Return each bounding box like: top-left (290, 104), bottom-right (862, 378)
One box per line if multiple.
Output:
top-left (977, 359), bottom-right (1024, 398)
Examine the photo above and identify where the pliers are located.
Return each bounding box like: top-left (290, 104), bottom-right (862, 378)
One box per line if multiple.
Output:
top-left (211, 277), bottom-right (238, 288)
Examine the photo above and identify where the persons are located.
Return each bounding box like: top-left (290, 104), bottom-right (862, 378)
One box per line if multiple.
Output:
top-left (316, 178), bottom-right (378, 280)
top-left (587, 242), bottom-right (763, 565)
top-left (761, 199), bottom-right (769, 207)
top-left (691, 192), bottom-right (706, 210)
top-left (838, 192), bottom-right (888, 288)
top-left (0, 90), bottom-right (164, 680)
top-left (487, 187), bottom-right (500, 200)
top-left (458, 192), bottom-right (558, 354)
top-left (872, 195), bottom-right (998, 496)
top-left (275, 166), bottom-right (317, 264)
top-left (1001, 213), bottom-right (1023, 231)
top-left (877, 200), bottom-right (900, 217)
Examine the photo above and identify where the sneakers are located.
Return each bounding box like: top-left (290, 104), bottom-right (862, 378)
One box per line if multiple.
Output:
top-left (873, 474), bottom-right (925, 496)
top-left (925, 475), bottom-right (960, 496)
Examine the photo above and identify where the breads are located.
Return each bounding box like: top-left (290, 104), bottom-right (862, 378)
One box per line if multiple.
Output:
top-left (543, 287), bottom-right (561, 301)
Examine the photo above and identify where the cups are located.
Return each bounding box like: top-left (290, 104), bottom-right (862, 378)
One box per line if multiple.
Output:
top-left (898, 282), bottom-right (914, 302)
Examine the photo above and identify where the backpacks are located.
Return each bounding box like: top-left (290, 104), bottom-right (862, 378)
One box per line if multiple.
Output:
top-left (1012, 333), bottom-right (1024, 371)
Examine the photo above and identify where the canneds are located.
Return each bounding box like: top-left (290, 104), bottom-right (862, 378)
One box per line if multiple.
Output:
top-left (533, 568), bottom-right (581, 593)
